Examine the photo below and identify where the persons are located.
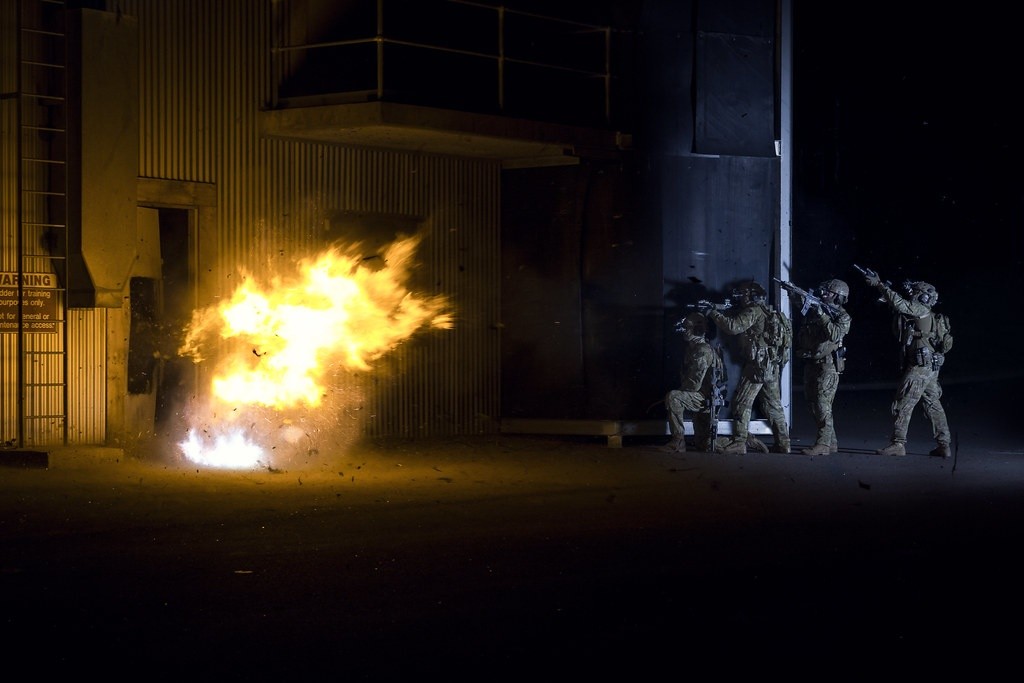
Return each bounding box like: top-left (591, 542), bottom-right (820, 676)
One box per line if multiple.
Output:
top-left (696, 281), bottom-right (791, 455)
top-left (658, 311), bottom-right (769, 455)
top-left (787, 278), bottom-right (852, 456)
top-left (866, 271), bottom-right (953, 457)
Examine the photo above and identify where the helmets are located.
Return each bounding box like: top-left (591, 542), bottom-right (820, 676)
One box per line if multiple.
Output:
top-left (734, 282), bottom-right (767, 302)
top-left (819, 278), bottom-right (849, 297)
top-left (910, 282), bottom-right (939, 307)
top-left (686, 312), bottom-right (706, 334)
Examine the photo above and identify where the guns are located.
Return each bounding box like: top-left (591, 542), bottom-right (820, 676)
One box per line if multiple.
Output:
top-left (773, 277), bottom-right (841, 319)
top-left (854, 263), bottom-right (907, 298)
top-left (686, 300), bottom-right (738, 309)
top-left (707, 367), bottom-right (729, 452)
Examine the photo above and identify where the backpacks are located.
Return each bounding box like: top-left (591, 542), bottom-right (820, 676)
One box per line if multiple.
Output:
top-left (745, 304), bottom-right (793, 348)
top-left (905, 306), bottom-right (953, 353)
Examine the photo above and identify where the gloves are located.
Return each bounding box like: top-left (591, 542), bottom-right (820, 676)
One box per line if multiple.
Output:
top-left (866, 270), bottom-right (881, 287)
top-left (703, 307), bottom-right (714, 315)
top-left (815, 306), bottom-right (823, 316)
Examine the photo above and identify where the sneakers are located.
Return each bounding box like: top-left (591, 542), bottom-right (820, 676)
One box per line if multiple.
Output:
top-left (801, 443), bottom-right (830, 456)
top-left (716, 441), bottom-right (747, 454)
top-left (660, 437), bottom-right (686, 453)
top-left (746, 432), bottom-right (770, 454)
top-left (767, 445), bottom-right (791, 454)
top-left (829, 445), bottom-right (838, 453)
top-left (877, 443), bottom-right (905, 456)
top-left (930, 447), bottom-right (951, 457)
